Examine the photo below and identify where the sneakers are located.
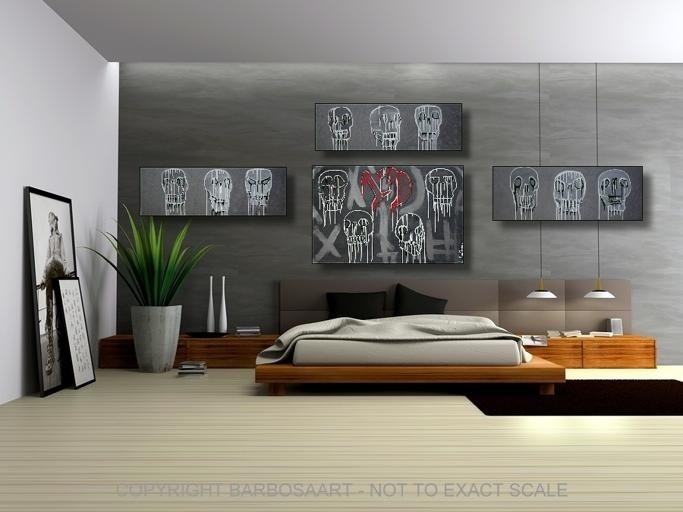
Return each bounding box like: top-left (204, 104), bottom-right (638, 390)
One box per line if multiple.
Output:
top-left (45, 357), bottom-right (55, 375)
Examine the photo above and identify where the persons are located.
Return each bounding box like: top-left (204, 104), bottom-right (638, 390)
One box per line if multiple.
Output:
top-left (41, 212), bottom-right (70, 375)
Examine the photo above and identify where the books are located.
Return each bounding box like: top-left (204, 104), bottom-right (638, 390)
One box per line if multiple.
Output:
top-left (236, 325), bottom-right (261, 336)
top-left (177, 361), bottom-right (208, 377)
top-left (545, 330), bottom-right (613, 339)
